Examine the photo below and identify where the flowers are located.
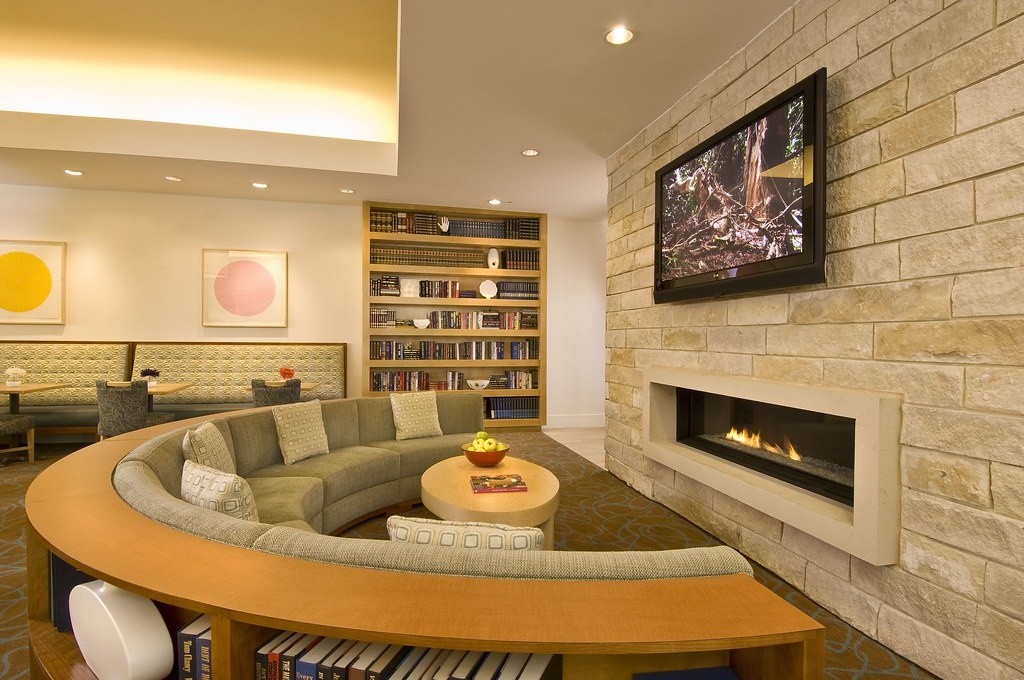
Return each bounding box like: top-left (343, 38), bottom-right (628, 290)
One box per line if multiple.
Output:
top-left (279, 368), bottom-right (294, 381)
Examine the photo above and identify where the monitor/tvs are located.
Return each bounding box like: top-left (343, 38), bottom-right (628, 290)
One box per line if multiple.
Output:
top-left (654, 67), bottom-right (828, 304)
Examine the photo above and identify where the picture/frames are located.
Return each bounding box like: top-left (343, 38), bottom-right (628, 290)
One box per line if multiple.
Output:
top-left (202, 248), bottom-right (288, 329)
top-left (0, 239), bottom-right (66, 326)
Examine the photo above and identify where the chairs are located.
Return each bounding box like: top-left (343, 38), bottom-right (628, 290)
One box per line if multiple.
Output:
top-left (95, 380), bottom-right (175, 441)
top-left (251, 378), bottom-right (301, 407)
top-left (0, 414), bottom-right (35, 464)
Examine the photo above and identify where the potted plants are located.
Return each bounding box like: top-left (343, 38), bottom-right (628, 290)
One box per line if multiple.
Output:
top-left (4, 368), bottom-right (27, 386)
top-left (140, 368), bottom-right (159, 387)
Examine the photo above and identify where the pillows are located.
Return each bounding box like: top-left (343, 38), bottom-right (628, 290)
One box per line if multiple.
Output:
top-left (386, 515), bottom-right (543, 551)
top-left (389, 390), bottom-right (443, 441)
top-left (272, 398), bottom-right (329, 466)
top-left (181, 459), bottom-right (260, 524)
top-left (181, 421), bottom-right (236, 476)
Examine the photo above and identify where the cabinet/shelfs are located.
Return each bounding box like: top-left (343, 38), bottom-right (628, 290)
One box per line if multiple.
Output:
top-left (360, 201), bottom-right (548, 426)
top-left (23, 408), bottom-right (826, 680)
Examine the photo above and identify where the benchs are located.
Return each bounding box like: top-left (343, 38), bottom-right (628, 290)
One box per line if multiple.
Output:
top-left (0, 340), bottom-right (132, 441)
top-left (131, 342), bottom-right (347, 416)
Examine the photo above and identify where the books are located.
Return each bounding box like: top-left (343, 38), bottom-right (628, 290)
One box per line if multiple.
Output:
top-left (370, 244), bottom-right (487, 268)
top-left (486, 397), bottom-right (539, 418)
top-left (427, 309), bottom-right (538, 330)
top-left (177, 613), bottom-right (211, 680)
top-left (370, 370), bottom-right (464, 392)
top-left (448, 218), bottom-right (539, 240)
top-left (470, 473), bottom-right (528, 494)
top-left (370, 211), bottom-right (442, 235)
top-left (370, 337), bottom-right (539, 360)
top-left (254, 631), bottom-right (554, 680)
top-left (370, 275), bottom-right (476, 298)
top-left (370, 308), bottom-right (414, 328)
top-left (488, 370), bottom-right (538, 389)
top-left (496, 281), bottom-right (539, 300)
top-left (500, 249), bottom-right (539, 270)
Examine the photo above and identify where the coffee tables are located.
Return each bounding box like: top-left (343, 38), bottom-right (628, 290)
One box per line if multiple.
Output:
top-left (420, 455), bottom-right (560, 551)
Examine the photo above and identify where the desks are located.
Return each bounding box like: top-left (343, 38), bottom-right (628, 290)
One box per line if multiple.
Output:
top-left (0, 383), bottom-right (71, 414)
top-left (107, 381), bottom-right (194, 413)
top-left (246, 381), bottom-right (321, 391)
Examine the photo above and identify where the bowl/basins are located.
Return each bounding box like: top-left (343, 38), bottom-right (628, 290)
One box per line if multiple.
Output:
top-left (462, 441), bottom-right (511, 467)
top-left (467, 380), bottom-right (490, 390)
top-left (412, 319), bottom-right (431, 329)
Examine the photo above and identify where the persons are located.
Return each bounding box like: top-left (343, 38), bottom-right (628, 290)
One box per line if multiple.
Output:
top-left (437, 216), bottom-right (449, 232)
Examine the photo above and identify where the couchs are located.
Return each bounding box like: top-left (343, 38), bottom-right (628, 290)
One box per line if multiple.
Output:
top-left (114, 393), bottom-right (752, 580)
